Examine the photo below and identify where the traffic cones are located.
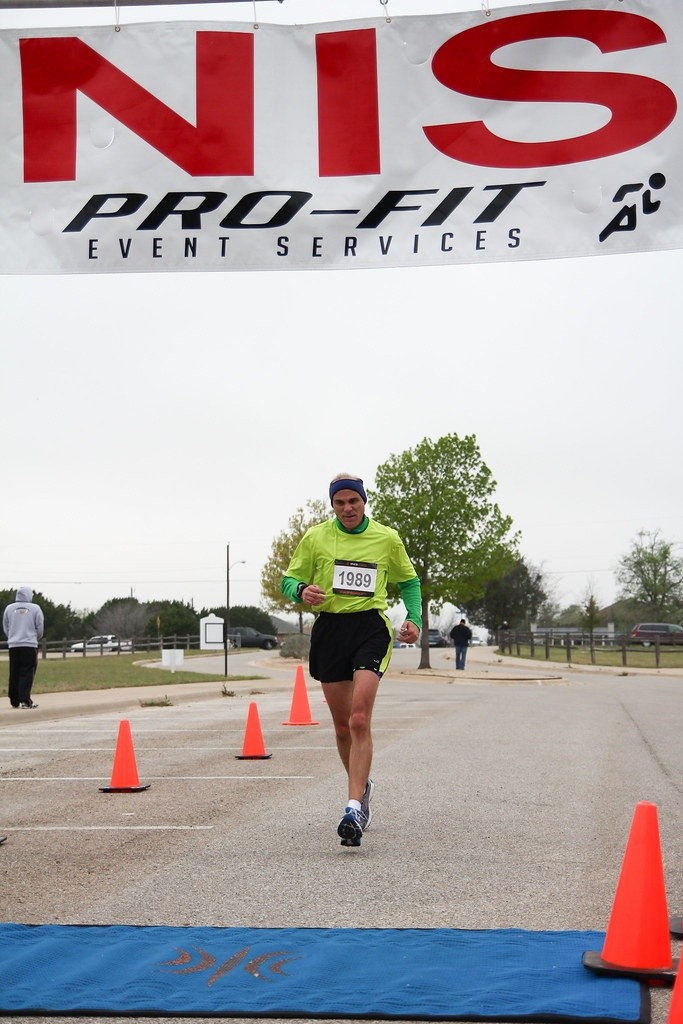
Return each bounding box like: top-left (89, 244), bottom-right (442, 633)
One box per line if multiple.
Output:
top-left (234, 702), bottom-right (273, 760)
top-left (663, 944), bottom-right (683, 1024)
top-left (279, 663), bottom-right (321, 726)
top-left (98, 720), bottom-right (152, 791)
top-left (580, 799), bottom-right (682, 982)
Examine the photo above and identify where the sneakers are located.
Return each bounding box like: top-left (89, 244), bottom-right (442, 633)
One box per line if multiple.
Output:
top-left (337, 806), bottom-right (368, 847)
top-left (360, 779), bottom-right (375, 831)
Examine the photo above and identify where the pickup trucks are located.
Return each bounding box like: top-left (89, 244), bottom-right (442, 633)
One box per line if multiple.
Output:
top-left (70, 634), bottom-right (133, 653)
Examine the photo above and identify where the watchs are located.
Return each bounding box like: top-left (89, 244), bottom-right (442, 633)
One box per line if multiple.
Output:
top-left (296, 584), bottom-right (306, 596)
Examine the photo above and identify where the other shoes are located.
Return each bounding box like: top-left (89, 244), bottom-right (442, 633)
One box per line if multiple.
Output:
top-left (21, 701), bottom-right (39, 709)
top-left (12, 704), bottom-right (19, 709)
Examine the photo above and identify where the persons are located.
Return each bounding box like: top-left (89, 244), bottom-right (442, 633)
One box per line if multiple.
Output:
top-left (2, 587), bottom-right (44, 708)
top-left (500, 620), bottom-right (508, 630)
top-left (280, 474), bottom-right (422, 848)
top-left (450, 619), bottom-right (472, 670)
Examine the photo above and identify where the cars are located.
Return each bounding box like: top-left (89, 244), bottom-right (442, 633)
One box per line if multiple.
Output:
top-left (399, 642), bottom-right (416, 648)
top-left (467, 636), bottom-right (484, 647)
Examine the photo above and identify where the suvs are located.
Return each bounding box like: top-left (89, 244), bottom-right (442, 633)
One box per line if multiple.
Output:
top-left (227, 625), bottom-right (278, 650)
top-left (630, 622), bottom-right (683, 647)
top-left (419, 628), bottom-right (450, 647)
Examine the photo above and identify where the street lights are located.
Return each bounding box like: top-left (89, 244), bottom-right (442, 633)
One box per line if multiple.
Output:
top-left (226, 542), bottom-right (247, 639)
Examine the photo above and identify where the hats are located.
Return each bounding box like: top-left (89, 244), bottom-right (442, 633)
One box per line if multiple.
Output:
top-left (461, 619), bottom-right (465, 623)
